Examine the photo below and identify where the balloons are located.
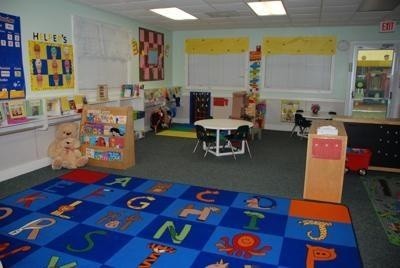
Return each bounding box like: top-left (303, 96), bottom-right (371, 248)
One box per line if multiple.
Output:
top-left (336, 39), bottom-right (351, 52)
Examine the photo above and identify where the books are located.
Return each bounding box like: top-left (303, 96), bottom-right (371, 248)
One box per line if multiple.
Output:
top-left (345, 147), bottom-right (371, 172)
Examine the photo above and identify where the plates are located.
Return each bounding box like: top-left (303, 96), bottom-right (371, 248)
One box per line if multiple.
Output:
top-left (302, 120), bottom-right (348, 204)
top-left (335, 115), bottom-right (400, 174)
top-left (85, 96), bottom-right (145, 143)
top-left (351, 59), bottom-right (392, 113)
top-left (231, 91), bottom-right (248, 119)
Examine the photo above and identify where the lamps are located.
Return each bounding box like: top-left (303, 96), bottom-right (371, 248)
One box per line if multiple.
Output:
top-left (289, 109), bottom-right (337, 142)
top-left (193, 113), bottom-right (265, 162)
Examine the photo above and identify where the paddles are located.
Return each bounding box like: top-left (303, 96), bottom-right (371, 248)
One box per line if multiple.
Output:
top-left (154, 124), bottom-right (228, 141)
top-left (0, 167), bottom-right (364, 267)
top-left (360, 172), bottom-right (400, 247)
